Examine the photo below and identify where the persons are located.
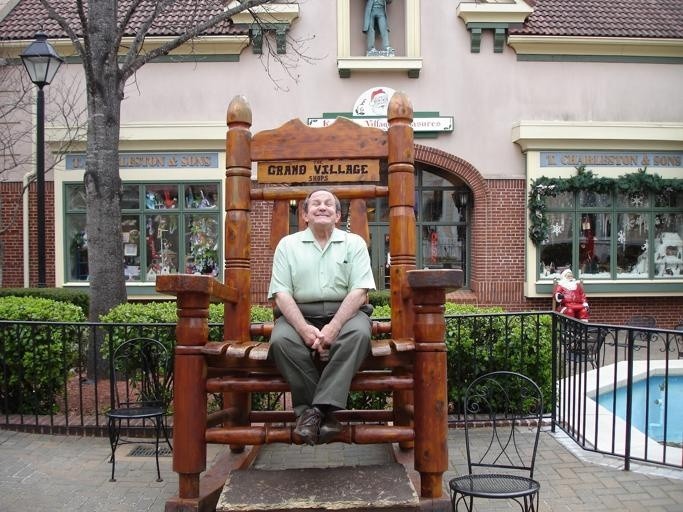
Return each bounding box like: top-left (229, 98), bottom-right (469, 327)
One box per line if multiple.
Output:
top-left (360, 0), bottom-right (396, 56)
top-left (549, 267), bottom-right (590, 342)
top-left (266, 188), bottom-right (377, 446)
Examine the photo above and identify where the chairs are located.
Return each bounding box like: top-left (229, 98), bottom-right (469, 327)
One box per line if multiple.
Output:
top-left (448, 370), bottom-right (545, 512)
top-left (551, 278), bottom-right (583, 339)
top-left (556, 314), bottom-right (683, 369)
top-left (104, 336), bottom-right (173, 483)
top-left (155, 89), bottom-right (454, 512)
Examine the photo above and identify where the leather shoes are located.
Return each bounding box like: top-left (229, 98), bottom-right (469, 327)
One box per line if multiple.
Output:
top-left (292, 407), bottom-right (342, 445)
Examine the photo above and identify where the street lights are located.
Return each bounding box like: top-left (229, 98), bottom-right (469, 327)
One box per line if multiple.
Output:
top-left (18, 34), bottom-right (65, 287)
